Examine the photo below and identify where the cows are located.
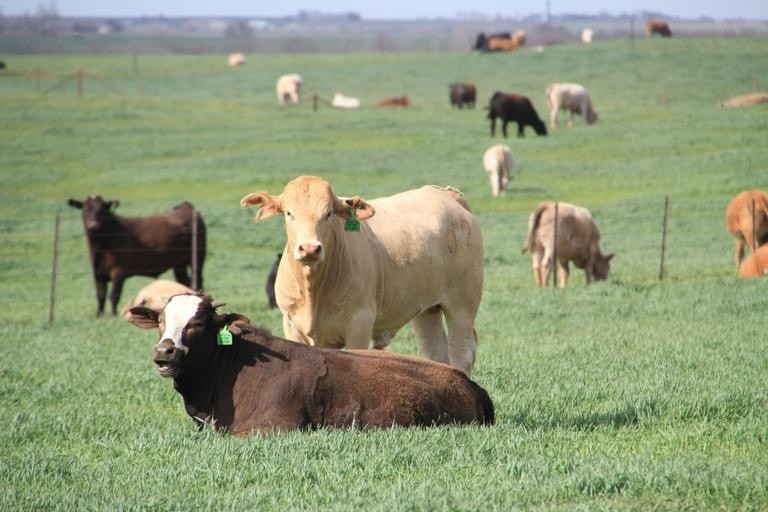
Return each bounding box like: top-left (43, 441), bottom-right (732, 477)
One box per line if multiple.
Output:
top-left (482, 145), bottom-right (515, 197)
top-left (520, 202), bottom-right (616, 290)
top-left (447, 82), bottom-right (476, 109)
top-left (649, 20), bottom-right (670, 36)
top-left (125, 289), bottom-right (495, 438)
top-left (482, 83), bottom-right (599, 137)
top-left (473, 31), bottom-right (525, 52)
top-left (726, 189), bottom-right (768, 278)
top-left (240, 175), bottom-right (484, 381)
top-left (722, 93), bottom-right (768, 108)
top-left (67, 195), bottom-right (206, 318)
